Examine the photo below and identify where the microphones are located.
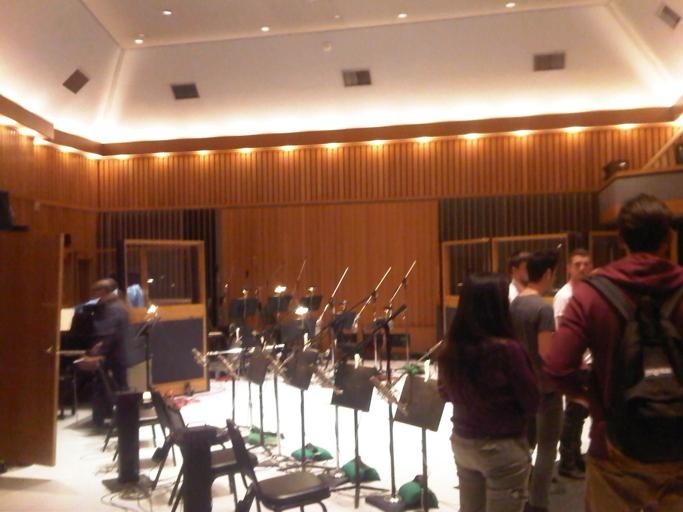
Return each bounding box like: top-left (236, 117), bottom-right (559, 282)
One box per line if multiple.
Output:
top-left (370, 376), bottom-right (413, 419)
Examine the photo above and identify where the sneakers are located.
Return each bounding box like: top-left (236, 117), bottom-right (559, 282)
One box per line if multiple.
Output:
top-left (558, 461), bottom-right (586, 480)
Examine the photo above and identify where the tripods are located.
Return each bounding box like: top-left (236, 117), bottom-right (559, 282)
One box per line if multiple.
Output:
top-left (214, 368), bottom-right (388, 510)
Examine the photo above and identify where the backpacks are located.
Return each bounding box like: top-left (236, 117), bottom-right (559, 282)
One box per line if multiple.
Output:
top-left (580, 271), bottom-right (681, 466)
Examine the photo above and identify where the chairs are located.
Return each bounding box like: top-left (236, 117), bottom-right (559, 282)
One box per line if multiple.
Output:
top-left (148, 385), bottom-right (332, 512)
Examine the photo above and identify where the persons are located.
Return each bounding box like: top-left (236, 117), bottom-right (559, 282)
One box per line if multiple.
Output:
top-left (73, 273), bottom-right (147, 431)
